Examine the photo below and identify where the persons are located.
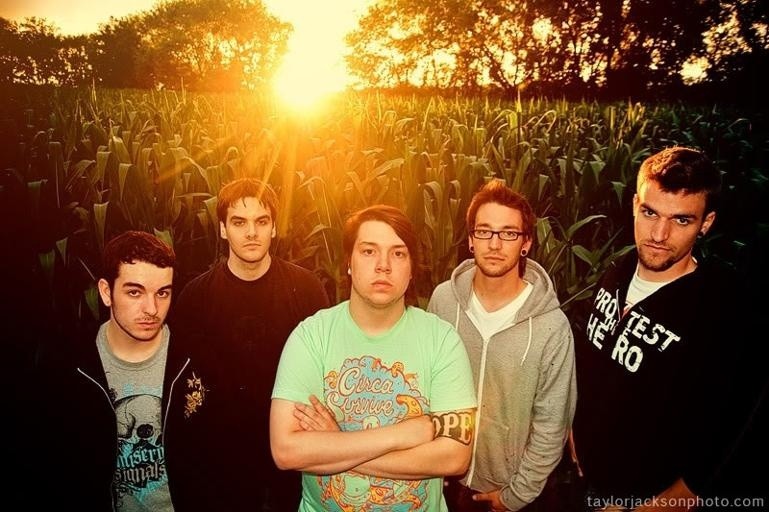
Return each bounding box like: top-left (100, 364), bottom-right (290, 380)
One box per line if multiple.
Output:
top-left (34, 231), bottom-right (229, 512)
top-left (172, 178), bottom-right (331, 512)
top-left (555, 147), bottom-right (762, 512)
top-left (270, 204), bottom-right (478, 512)
top-left (425, 179), bottom-right (577, 512)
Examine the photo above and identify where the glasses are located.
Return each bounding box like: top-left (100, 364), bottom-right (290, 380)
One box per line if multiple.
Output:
top-left (469, 230), bottom-right (525, 240)
